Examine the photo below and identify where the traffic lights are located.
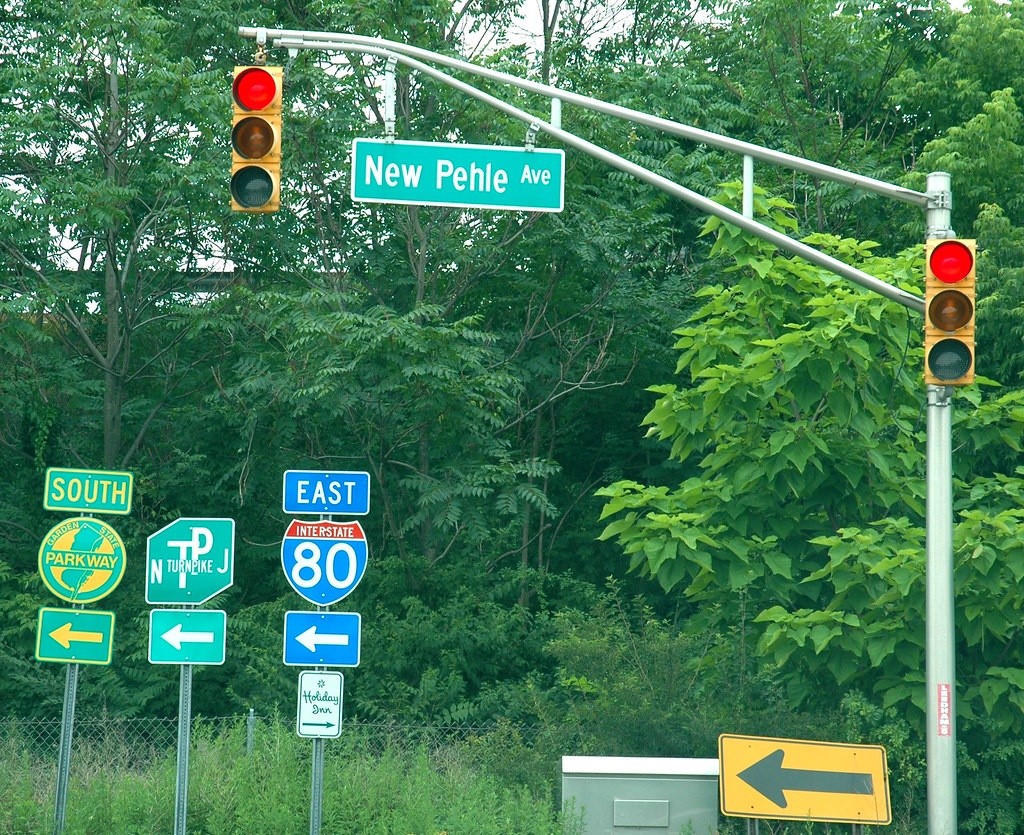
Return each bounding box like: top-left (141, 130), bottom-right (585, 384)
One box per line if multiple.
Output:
top-left (231, 65), bottom-right (283, 212)
top-left (925, 240), bottom-right (977, 385)
